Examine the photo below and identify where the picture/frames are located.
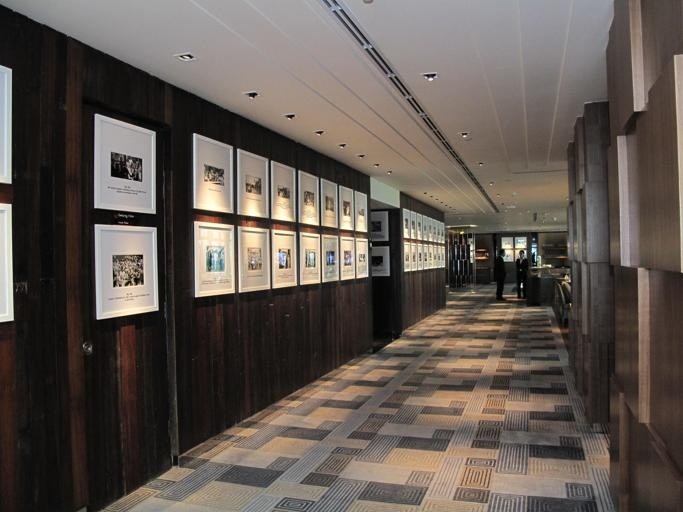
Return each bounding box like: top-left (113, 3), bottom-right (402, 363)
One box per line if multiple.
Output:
top-left (80, 95), bottom-right (171, 333)
top-left (369, 209), bottom-right (392, 279)
top-left (0, 66), bottom-right (14, 324)
top-left (402, 207), bottom-right (445, 274)
top-left (186, 130), bottom-right (370, 301)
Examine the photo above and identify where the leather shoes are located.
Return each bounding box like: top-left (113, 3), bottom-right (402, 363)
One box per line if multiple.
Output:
top-left (496, 296), bottom-right (506, 301)
top-left (517, 296), bottom-right (526, 299)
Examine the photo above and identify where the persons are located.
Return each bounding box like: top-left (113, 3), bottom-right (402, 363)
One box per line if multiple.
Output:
top-left (492, 249), bottom-right (508, 302)
top-left (514, 249), bottom-right (528, 299)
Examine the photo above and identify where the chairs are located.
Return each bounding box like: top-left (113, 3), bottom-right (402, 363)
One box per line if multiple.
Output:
top-left (554, 277), bottom-right (571, 328)
